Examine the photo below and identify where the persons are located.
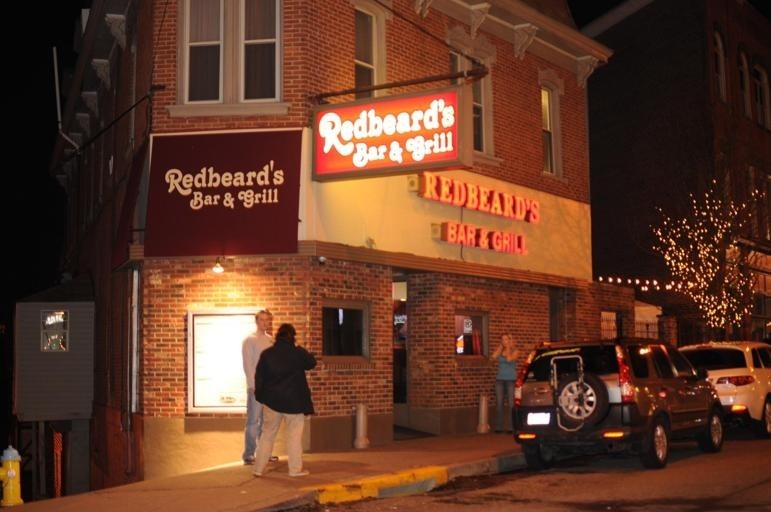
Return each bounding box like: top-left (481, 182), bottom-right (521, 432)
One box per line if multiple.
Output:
top-left (488, 332), bottom-right (520, 433)
top-left (253, 322), bottom-right (316, 477)
top-left (242, 309), bottom-right (281, 465)
top-left (394, 322), bottom-right (408, 346)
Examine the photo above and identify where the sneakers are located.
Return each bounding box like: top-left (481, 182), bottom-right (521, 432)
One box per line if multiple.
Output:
top-left (289, 470), bottom-right (309, 477)
top-left (252, 471), bottom-right (262, 477)
top-left (268, 456), bottom-right (278, 464)
top-left (244, 459), bottom-right (256, 465)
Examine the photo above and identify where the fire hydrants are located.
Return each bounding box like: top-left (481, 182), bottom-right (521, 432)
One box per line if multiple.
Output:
top-left (0, 445), bottom-right (26, 507)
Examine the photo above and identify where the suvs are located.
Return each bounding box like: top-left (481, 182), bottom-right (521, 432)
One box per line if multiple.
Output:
top-left (677, 341), bottom-right (770, 440)
top-left (510, 336), bottom-right (724, 468)
